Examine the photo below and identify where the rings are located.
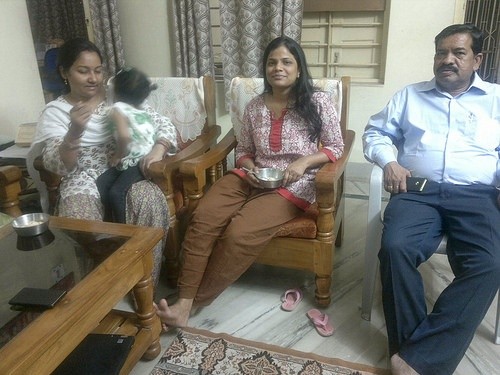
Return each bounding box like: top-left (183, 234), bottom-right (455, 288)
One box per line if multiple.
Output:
top-left (394, 186), bottom-right (399, 188)
top-left (388, 186), bottom-right (393, 189)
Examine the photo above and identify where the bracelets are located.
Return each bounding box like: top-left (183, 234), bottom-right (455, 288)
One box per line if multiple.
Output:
top-left (156, 141), bottom-right (169, 149)
top-left (63, 137), bottom-right (81, 149)
top-left (67, 122), bottom-right (83, 139)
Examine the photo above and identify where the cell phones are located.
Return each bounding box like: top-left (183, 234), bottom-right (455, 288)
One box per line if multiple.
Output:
top-left (9, 287), bottom-right (66, 308)
top-left (406, 177), bottom-right (427, 192)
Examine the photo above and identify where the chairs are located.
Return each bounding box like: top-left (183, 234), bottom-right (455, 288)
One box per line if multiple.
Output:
top-left (356, 161), bottom-right (500, 345)
top-left (0, 165), bottom-right (23, 229)
top-left (31, 74), bottom-right (222, 286)
top-left (179, 75), bottom-right (355, 309)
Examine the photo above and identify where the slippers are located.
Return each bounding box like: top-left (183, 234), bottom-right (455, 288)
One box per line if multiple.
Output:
top-left (282, 287), bottom-right (303, 311)
top-left (307, 309), bottom-right (334, 336)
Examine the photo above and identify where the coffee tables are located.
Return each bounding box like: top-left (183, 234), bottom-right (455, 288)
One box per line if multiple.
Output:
top-left (0, 216), bottom-right (166, 375)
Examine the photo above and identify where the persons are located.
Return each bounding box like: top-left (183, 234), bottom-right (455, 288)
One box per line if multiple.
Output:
top-left (26, 37), bottom-right (178, 301)
top-left (363, 24), bottom-right (500, 375)
top-left (152, 36), bottom-right (344, 333)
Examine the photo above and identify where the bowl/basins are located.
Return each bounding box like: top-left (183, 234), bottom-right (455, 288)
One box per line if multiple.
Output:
top-left (12, 213), bottom-right (51, 237)
top-left (256, 167), bottom-right (284, 188)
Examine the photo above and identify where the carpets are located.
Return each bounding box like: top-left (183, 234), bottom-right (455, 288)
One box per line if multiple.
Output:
top-left (94, 312), bottom-right (393, 375)
